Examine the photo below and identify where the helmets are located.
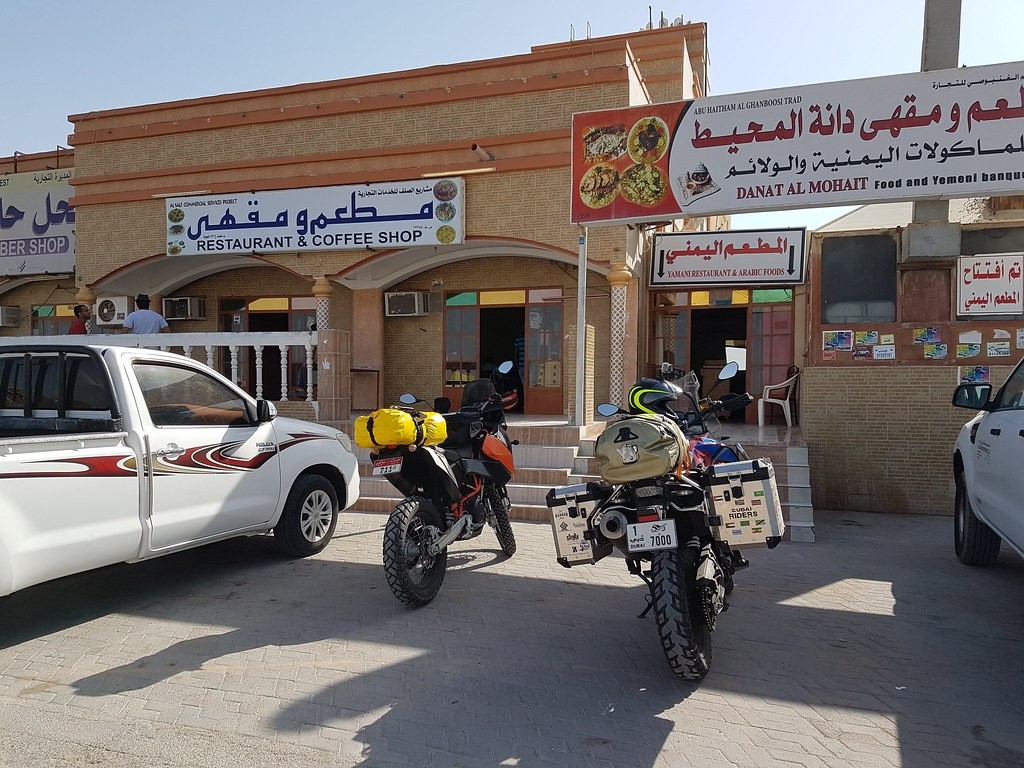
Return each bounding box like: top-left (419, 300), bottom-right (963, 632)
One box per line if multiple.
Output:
top-left (626, 377), bottom-right (683, 423)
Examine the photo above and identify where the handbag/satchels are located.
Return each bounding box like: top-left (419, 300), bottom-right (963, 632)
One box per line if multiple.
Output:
top-left (594, 414), bottom-right (691, 484)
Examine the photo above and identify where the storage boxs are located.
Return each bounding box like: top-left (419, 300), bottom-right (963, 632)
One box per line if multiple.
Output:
top-left (546, 480), bottom-right (614, 568)
top-left (709, 456), bottom-right (786, 547)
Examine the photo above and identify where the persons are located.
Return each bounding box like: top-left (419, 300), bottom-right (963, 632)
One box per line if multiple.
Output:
top-left (68, 304), bottom-right (91, 334)
top-left (121, 293), bottom-right (172, 350)
top-left (301, 323), bottom-right (316, 399)
top-left (514, 326), bottom-right (525, 409)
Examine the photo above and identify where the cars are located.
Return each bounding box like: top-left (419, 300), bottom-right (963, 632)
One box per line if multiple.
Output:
top-left (951, 356), bottom-right (1023, 562)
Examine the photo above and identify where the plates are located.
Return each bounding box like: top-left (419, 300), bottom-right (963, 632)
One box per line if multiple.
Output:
top-left (627, 117), bottom-right (670, 163)
top-left (168, 245), bottom-right (182, 255)
top-left (436, 225), bottom-right (455, 243)
top-left (621, 164), bottom-right (668, 207)
top-left (434, 179), bottom-right (458, 201)
top-left (168, 209), bottom-right (184, 222)
top-left (169, 225), bottom-right (185, 235)
top-left (579, 164), bottom-right (620, 209)
top-left (436, 202), bottom-right (456, 221)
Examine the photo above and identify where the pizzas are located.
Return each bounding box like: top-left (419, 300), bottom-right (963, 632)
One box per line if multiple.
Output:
top-left (579, 163), bottom-right (622, 209)
top-left (619, 164), bottom-right (669, 208)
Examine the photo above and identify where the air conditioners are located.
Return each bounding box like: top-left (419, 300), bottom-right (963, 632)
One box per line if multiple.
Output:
top-left (0, 305), bottom-right (19, 327)
top-left (385, 291), bottom-right (430, 317)
top-left (162, 297), bottom-right (207, 321)
top-left (96, 296), bottom-right (135, 325)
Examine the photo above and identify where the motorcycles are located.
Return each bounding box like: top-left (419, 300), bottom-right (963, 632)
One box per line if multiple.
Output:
top-left (369, 355), bottom-right (516, 607)
top-left (546, 360), bottom-right (785, 679)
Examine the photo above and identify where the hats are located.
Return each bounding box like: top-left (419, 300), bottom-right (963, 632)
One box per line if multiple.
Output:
top-left (134, 294), bottom-right (152, 302)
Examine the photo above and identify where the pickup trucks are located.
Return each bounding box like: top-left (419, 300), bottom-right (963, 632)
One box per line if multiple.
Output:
top-left (1, 344), bottom-right (360, 603)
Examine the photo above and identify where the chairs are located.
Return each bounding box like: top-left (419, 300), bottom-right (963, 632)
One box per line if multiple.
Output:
top-left (758, 366), bottom-right (802, 427)
